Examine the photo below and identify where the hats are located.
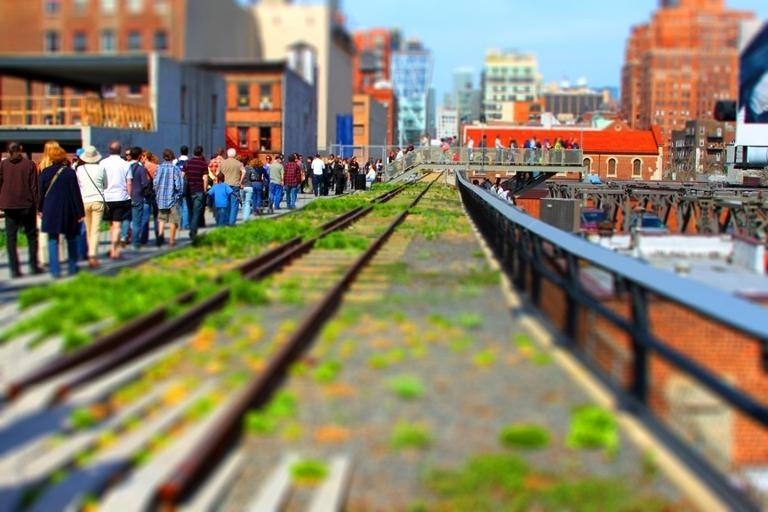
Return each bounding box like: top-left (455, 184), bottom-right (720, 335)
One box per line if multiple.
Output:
top-left (79, 145), bottom-right (102, 163)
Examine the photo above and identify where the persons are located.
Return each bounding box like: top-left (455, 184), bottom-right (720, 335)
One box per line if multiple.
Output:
top-left (387, 132), bottom-right (580, 180)
top-left (1, 140), bottom-right (382, 280)
top-left (473, 176), bottom-right (518, 205)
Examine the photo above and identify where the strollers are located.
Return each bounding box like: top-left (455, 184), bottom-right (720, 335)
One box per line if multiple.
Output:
top-left (250, 179), bottom-right (275, 215)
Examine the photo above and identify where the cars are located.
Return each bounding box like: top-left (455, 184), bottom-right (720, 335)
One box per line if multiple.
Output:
top-left (577, 205), bottom-right (616, 234)
top-left (626, 210), bottom-right (670, 236)
